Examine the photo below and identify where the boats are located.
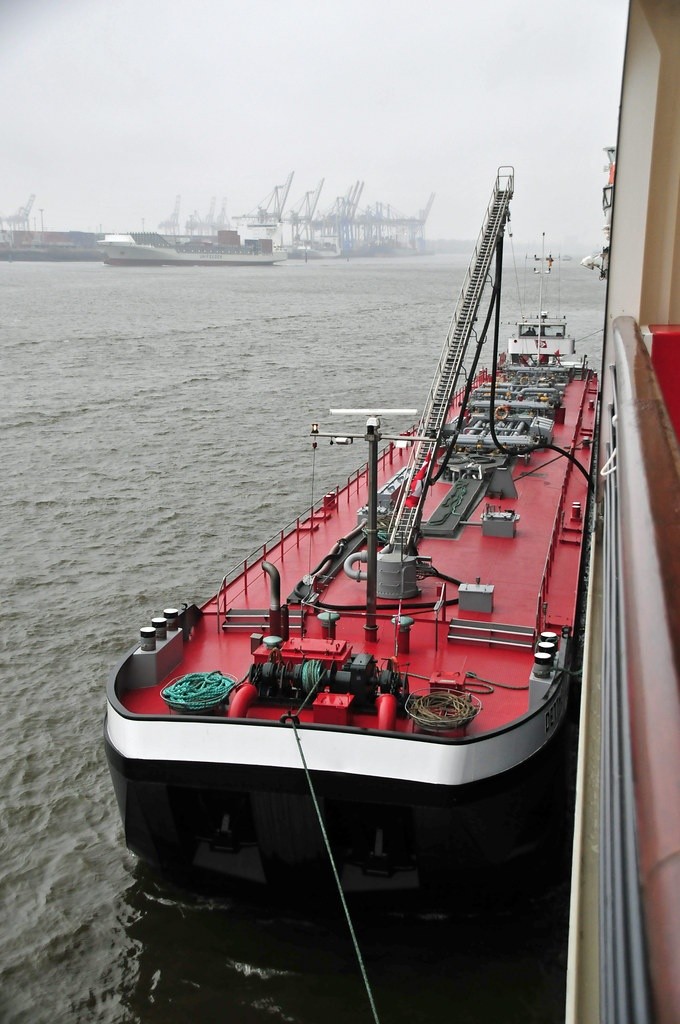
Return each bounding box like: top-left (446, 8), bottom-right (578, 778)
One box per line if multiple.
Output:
top-left (101, 162), bottom-right (600, 908)
top-left (96, 234), bottom-right (289, 269)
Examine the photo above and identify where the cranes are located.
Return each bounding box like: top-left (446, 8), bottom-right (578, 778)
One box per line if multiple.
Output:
top-left (162, 170), bottom-right (436, 253)
top-left (17, 193), bottom-right (37, 231)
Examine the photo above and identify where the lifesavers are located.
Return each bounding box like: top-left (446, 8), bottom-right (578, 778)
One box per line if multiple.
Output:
top-left (493, 406), bottom-right (509, 421)
